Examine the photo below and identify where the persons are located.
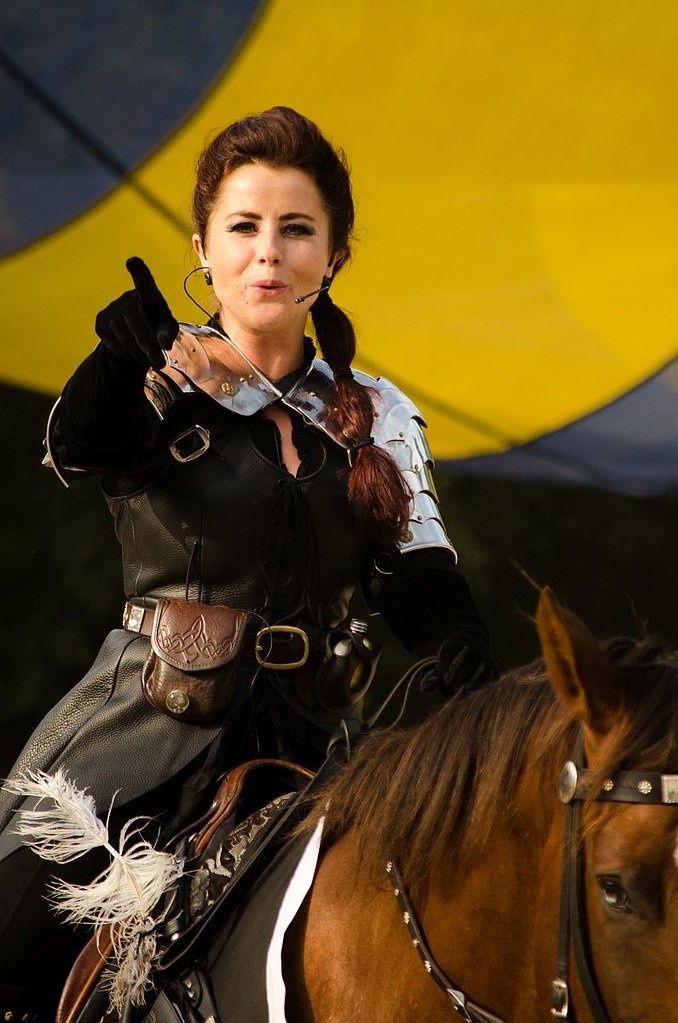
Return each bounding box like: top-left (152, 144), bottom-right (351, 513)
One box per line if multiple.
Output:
top-left (0, 106), bottom-right (520, 1023)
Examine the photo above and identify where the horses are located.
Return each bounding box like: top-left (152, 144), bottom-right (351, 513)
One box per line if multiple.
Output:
top-left (31, 584), bottom-right (678, 1022)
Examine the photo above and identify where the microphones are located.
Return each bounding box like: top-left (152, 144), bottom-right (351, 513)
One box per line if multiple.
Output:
top-left (294, 286), bottom-right (330, 303)
